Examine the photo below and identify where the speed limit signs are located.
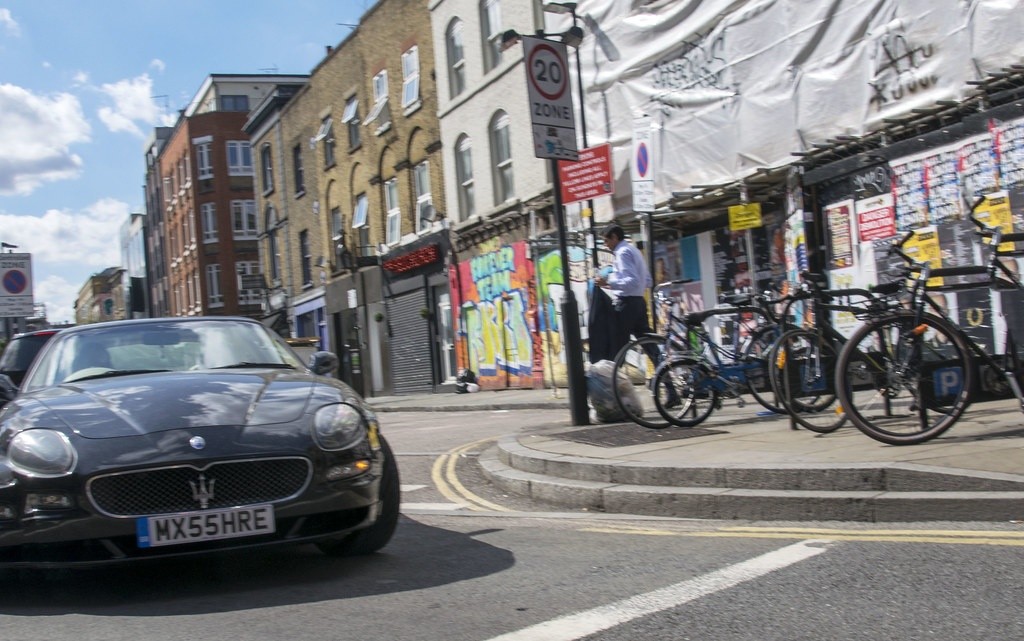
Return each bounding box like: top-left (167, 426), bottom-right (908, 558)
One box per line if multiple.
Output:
top-left (530, 44), bottom-right (569, 102)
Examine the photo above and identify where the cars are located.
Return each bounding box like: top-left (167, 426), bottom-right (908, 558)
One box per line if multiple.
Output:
top-left (0, 326), bottom-right (116, 409)
top-left (0, 314), bottom-right (400, 598)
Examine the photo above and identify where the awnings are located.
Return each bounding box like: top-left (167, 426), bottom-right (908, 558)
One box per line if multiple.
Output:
top-left (259, 310), bottom-right (284, 332)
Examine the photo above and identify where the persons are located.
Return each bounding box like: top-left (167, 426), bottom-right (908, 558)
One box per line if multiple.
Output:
top-left (594, 224), bottom-right (682, 408)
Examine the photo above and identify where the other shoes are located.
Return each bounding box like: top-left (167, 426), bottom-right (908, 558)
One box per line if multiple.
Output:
top-left (664, 399), bottom-right (683, 409)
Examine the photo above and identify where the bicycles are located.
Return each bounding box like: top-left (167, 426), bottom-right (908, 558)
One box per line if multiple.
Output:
top-left (612, 195), bottom-right (1021, 446)
